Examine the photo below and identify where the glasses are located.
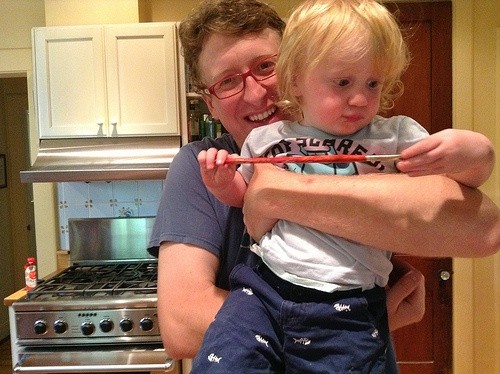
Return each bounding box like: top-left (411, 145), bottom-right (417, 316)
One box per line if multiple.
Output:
top-left (197, 54), bottom-right (277, 100)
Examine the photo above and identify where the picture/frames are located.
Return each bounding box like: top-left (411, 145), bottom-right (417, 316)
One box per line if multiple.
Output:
top-left (0, 154), bottom-right (8, 188)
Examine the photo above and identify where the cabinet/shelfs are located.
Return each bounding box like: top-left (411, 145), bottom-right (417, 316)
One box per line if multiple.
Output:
top-left (31, 22), bottom-right (182, 140)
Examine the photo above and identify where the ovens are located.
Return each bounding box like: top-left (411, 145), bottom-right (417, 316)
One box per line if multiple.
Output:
top-left (14, 349), bottom-right (180, 374)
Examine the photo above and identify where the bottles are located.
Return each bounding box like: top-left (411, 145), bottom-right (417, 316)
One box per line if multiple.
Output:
top-left (23, 257), bottom-right (37, 290)
top-left (188, 100), bottom-right (222, 142)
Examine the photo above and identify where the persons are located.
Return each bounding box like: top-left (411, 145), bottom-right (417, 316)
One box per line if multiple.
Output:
top-left (197, 0), bottom-right (496, 374)
top-left (148, 0), bottom-right (500, 357)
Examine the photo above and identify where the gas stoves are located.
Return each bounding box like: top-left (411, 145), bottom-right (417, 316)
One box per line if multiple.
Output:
top-left (12, 217), bottom-right (162, 348)
